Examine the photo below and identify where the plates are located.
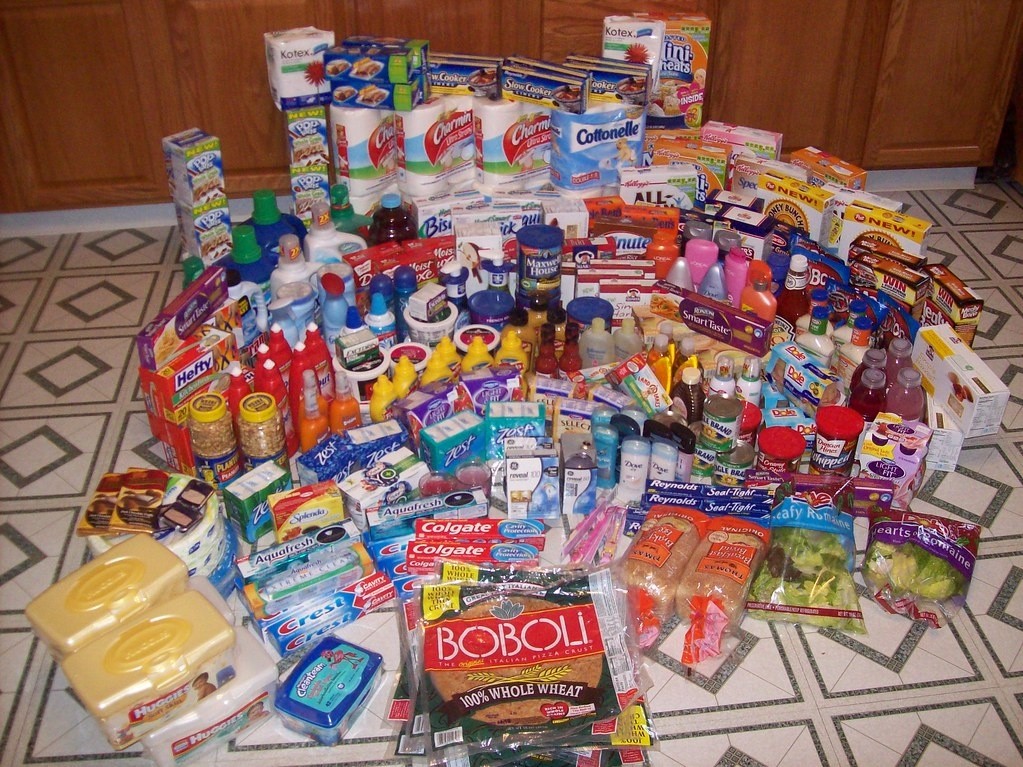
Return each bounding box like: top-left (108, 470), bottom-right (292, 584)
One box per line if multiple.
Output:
top-left (333, 86), bottom-right (358, 103)
top-left (325, 60), bottom-right (352, 76)
top-left (348, 60), bottom-right (384, 81)
top-left (355, 89), bottom-right (390, 108)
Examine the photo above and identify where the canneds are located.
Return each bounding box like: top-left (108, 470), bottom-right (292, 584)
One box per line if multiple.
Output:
top-left (687, 393), bottom-right (863, 488)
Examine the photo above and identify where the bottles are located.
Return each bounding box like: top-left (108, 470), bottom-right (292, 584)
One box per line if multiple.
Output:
top-left (183, 183), bottom-right (924, 486)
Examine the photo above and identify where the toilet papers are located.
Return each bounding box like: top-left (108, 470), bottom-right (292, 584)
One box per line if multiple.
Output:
top-left (328, 98), bottom-right (648, 201)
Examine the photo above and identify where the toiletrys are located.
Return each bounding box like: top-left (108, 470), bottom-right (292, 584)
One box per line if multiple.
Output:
top-left (226, 217), bottom-right (875, 658)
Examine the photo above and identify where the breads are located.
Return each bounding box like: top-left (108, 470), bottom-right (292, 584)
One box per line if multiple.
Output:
top-left (618, 510), bottom-right (766, 636)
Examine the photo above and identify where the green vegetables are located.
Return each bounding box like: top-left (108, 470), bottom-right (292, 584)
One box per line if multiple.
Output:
top-left (736, 519), bottom-right (977, 635)
